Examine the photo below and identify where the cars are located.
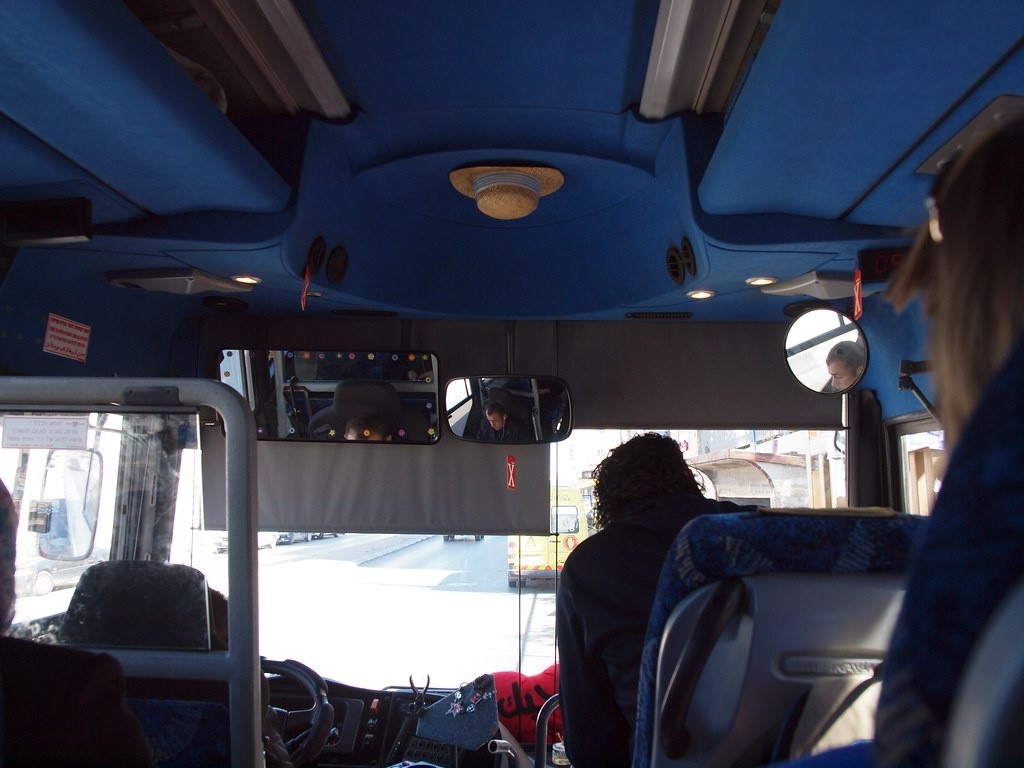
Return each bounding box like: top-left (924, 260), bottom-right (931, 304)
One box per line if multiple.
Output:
top-left (212, 531), bottom-right (346, 555)
top-left (15, 531), bottom-right (109, 597)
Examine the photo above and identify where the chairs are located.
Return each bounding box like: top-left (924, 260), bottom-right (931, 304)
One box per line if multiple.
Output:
top-left (58, 561), bottom-right (228, 768)
top-left (309, 380), bottom-right (429, 442)
top-left (483, 387), bottom-right (528, 424)
top-left (633, 506), bottom-right (929, 768)
top-left (769, 339), bottom-right (1024, 767)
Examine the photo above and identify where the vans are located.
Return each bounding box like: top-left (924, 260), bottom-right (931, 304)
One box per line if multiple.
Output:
top-left (508, 486), bottom-right (588, 587)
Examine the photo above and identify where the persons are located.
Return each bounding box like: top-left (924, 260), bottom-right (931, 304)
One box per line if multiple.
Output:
top-left (765, 112), bottom-right (1024, 768)
top-left (826, 341), bottom-right (866, 390)
top-left (477, 402), bottom-right (536, 441)
top-left (531, 379), bottom-right (566, 437)
top-left (343, 413), bottom-right (392, 440)
top-left (201, 588), bottom-right (293, 768)
top-left (0, 477), bottom-right (154, 768)
top-left (555, 433), bottom-right (767, 768)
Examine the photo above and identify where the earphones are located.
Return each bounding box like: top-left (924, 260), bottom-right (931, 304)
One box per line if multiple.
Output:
top-left (504, 417), bottom-right (505, 420)
